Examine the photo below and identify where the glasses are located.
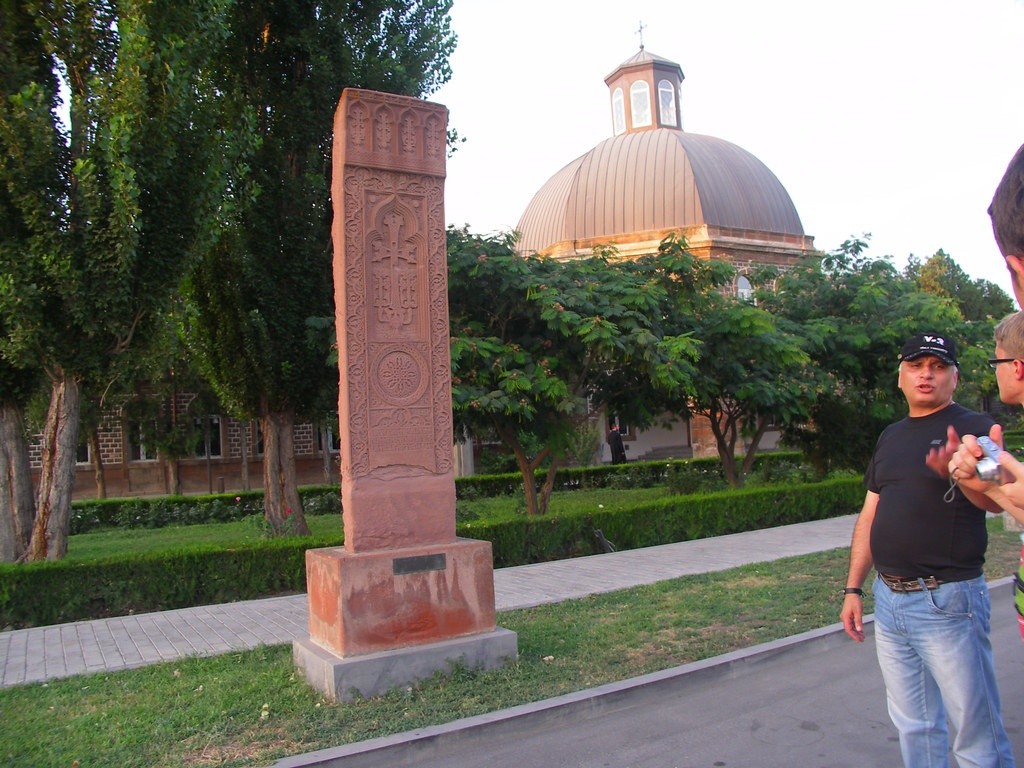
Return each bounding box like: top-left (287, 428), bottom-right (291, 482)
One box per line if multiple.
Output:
top-left (986, 356), bottom-right (1023, 371)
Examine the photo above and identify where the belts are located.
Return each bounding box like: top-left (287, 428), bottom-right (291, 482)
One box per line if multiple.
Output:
top-left (877, 570), bottom-right (945, 593)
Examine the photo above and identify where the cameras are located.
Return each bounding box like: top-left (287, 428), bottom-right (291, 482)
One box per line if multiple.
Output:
top-left (974, 436), bottom-right (1015, 487)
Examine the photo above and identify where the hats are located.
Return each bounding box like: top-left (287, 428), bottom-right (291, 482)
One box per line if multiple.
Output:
top-left (899, 331), bottom-right (959, 366)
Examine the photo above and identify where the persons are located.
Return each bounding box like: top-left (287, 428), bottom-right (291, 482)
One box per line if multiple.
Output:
top-left (608, 422), bottom-right (627, 465)
top-left (948, 145), bottom-right (1024, 642)
top-left (840, 334), bottom-right (1013, 768)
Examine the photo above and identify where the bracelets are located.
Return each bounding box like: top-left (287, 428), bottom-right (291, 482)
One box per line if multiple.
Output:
top-left (844, 588), bottom-right (867, 598)
